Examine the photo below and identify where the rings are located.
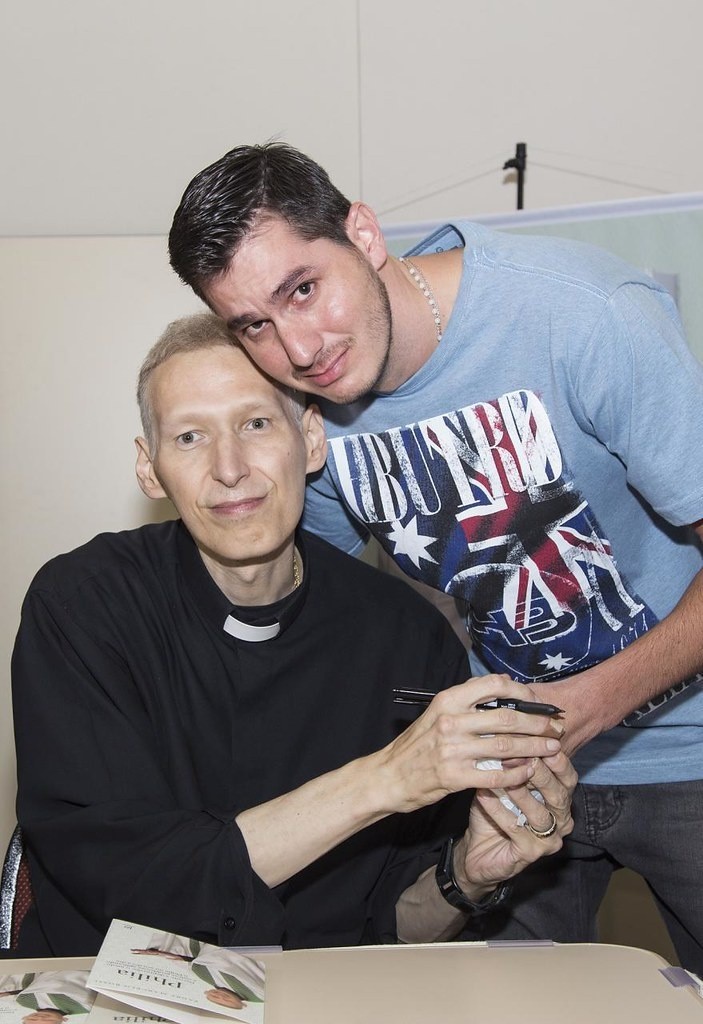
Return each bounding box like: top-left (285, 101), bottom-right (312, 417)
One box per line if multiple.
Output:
top-left (529, 811), bottom-right (558, 838)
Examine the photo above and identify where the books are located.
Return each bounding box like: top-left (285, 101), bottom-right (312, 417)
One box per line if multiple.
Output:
top-left (0, 971), bottom-right (99, 1024)
top-left (83, 919), bottom-right (267, 1024)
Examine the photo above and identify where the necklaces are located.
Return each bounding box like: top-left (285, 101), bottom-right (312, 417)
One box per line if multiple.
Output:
top-left (292, 556), bottom-right (302, 592)
top-left (396, 257), bottom-right (442, 343)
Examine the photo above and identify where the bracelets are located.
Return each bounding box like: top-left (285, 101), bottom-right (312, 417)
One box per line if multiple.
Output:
top-left (435, 837), bottom-right (516, 920)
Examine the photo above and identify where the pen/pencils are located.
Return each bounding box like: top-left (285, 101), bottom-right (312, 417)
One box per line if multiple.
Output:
top-left (391, 687), bottom-right (566, 716)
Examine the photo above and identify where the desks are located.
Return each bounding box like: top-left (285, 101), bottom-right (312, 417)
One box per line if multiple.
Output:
top-left (0, 941), bottom-right (703, 1024)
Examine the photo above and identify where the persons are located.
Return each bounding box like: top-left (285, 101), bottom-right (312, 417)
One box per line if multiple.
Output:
top-left (1, 973), bottom-right (98, 1024)
top-left (168, 143), bottom-right (703, 983)
top-left (131, 933), bottom-right (265, 1010)
top-left (10, 312), bottom-right (577, 958)
